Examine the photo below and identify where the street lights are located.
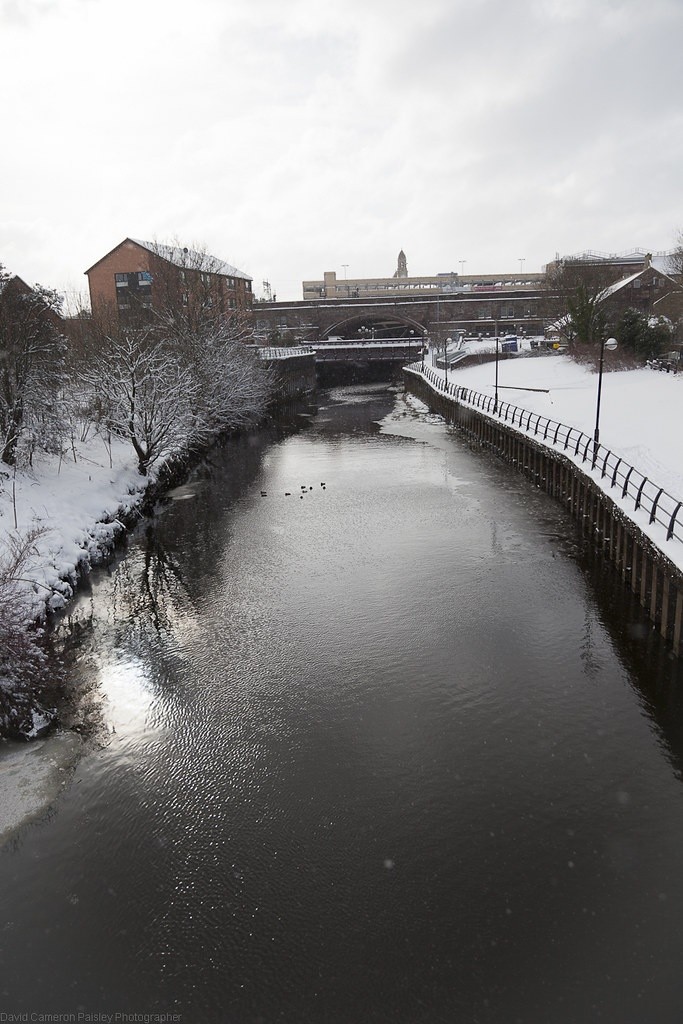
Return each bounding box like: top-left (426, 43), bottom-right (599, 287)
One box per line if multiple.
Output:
top-left (518, 259), bottom-right (525, 273)
top-left (459, 260), bottom-right (466, 275)
top-left (444, 338), bottom-right (452, 392)
top-left (342, 265), bottom-right (349, 279)
top-left (369, 328), bottom-right (376, 339)
top-left (520, 327), bottom-right (526, 348)
top-left (493, 338), bottom-right (506, 412)
top-left (421, 330), bottom-right (428, 372)
top-left (591, 337), bottom-right (618, 470)
top-left (409, 330), bottom-right (414, 359)
top-left (358, 326), bottom-right (368, 339)
